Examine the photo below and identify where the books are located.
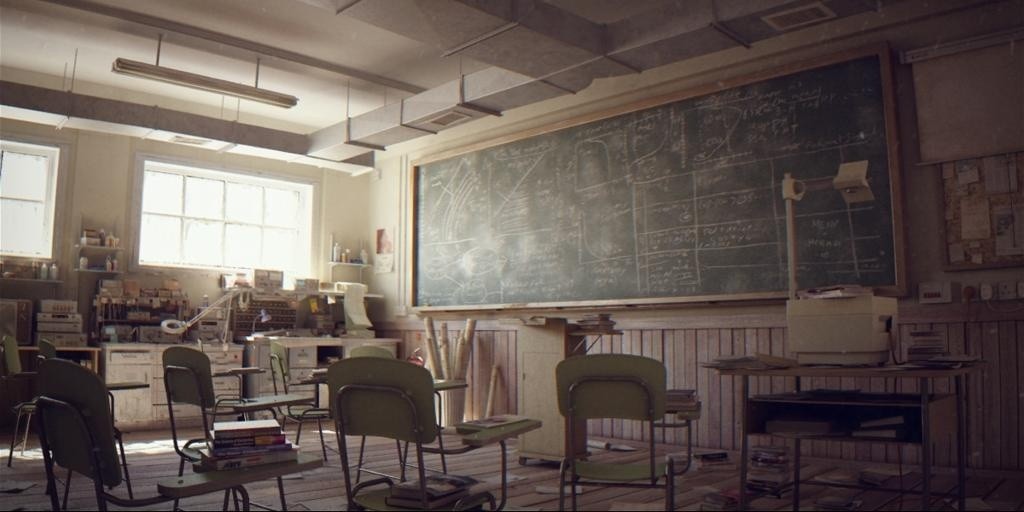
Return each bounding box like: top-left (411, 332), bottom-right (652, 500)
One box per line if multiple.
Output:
top-left (383, 488), bottom-right (469, 509)
top-left (703, 354), bottom-right (795, 370)
top-left (196, 416), bottom-right (301, 472)
top-left (945, 497), bottom-right (992, 510)
top-left (806, 286), bottom-right (873, 298)
top-left (391, 471), bottom-right (480, 497)
top-left (765, 417), bottom-right (905, 439)
top-left (666, 388), bottom-right (703, 421)
top-left (588, 439), bottom-right (638, 452)
top-left (695, 447), bottom-right (742, 511)
top-left (817, 465), bottom-right (913, 510)
top-left (746, 443), bottom-right (792, 499)
top-left (462, 415), bottom-right (528, 429)
top-left (577, 313), bottom-right (616, 331)
top-left (907, 331), bottom-right (978, 368)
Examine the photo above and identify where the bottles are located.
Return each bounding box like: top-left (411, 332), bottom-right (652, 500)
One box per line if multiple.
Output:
top-left (79, 255), bottom-right (88, 270)
top-left (31, 259), bottom-right (59, 281)
top-left (202, 294), bottom-right (209, 307)
top-left (332, 243), bottom-right (369, 265)
top-left (99, 228), bottom-right (120, 272)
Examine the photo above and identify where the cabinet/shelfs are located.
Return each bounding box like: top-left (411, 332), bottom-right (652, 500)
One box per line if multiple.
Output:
top-left (320, 234), bottom-right (382, 298)
top-left (70, 212), bottom-right (123, 275)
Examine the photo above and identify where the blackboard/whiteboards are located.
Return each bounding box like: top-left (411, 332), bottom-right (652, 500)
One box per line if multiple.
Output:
top-left (395, 42), bottom-right (910, 314)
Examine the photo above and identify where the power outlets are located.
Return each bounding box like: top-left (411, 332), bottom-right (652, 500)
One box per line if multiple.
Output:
top-left (996, 280), bottom-right (1017, 299)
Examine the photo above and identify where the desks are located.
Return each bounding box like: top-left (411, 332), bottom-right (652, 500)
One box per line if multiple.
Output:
top-left (716, 358), bottom-right (986, 512)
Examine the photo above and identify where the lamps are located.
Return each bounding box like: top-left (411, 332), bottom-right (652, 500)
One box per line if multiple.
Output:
top-left (108, 32), bottom-right (299, 112)
